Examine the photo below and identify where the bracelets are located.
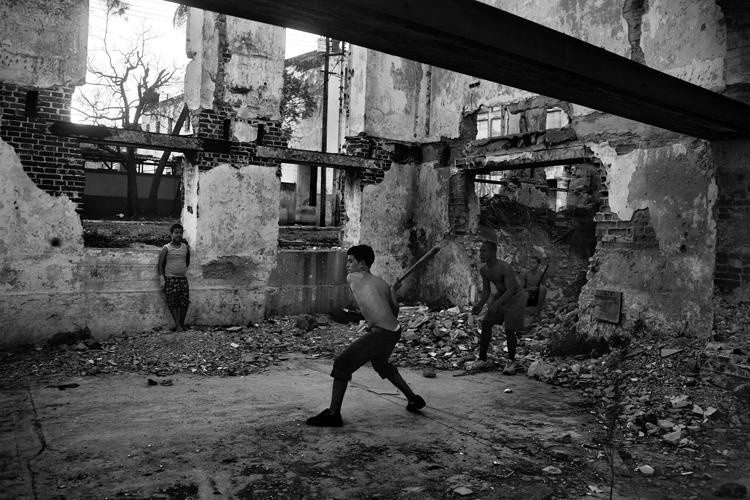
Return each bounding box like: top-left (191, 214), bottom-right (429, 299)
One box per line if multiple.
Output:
top-left (160, 274), bottom-right (164, 281)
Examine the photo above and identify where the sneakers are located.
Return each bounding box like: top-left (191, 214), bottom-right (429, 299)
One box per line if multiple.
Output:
top-left (306, 408), bottom-right (344, 428)
top-left (406, 395), bottom-right (427, 412)
top-left (464, 358), bottom-right (489, 368)
top-left (503, 359), bottom-right (518, 376)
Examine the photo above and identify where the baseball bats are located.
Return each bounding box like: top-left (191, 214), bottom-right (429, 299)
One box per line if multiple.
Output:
top-left (389, 245), bottom-right (442, 289)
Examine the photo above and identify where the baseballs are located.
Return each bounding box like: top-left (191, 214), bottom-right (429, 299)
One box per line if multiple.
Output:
top-left (51, 237), bottom-right (61, 247)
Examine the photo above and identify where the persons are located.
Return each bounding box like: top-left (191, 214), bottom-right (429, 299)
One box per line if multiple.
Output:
top-left (159, 223), bottom-right (190, 332)
top-left (308, 244), bottom-right (427, 428)
top-left (520, 256), bottom-right (547, 313)
top-left (467, 238), bottom-right (527, 376)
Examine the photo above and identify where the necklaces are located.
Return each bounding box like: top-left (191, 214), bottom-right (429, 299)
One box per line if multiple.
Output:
top-left (171, 243), bottom-right (181, 248)
